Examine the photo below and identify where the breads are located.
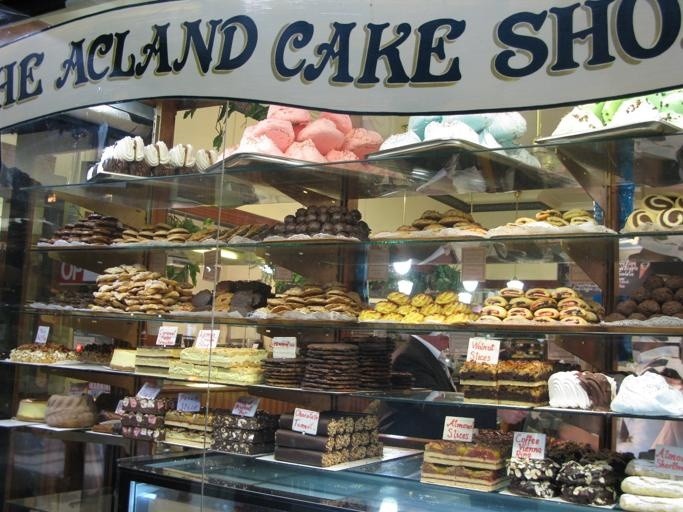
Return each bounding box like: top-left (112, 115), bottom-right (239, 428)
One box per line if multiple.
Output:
top-left (10, 88), bottom-right (683, 512)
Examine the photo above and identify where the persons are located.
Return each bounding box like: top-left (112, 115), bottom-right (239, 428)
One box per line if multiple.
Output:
top-left (379, 325), bottom-right (461, 437)
